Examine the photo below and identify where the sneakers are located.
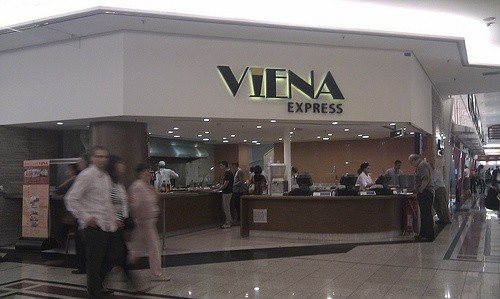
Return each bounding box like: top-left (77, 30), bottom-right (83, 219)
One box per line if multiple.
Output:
top-left (221, 224), bottom-right (232, 229)
top-left (436, 218), bottom-right (452, 224)
top-left (414, 234), bottom-right (433, 242)
top-left (72, 269), bottom-right (87, 274)
top-left (150, 273), bottom-right (171, 283)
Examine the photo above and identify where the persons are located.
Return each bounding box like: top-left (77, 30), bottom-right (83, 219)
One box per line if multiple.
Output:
top-left (409, 153), bottom-right (435, 242)
top-left (248, 166), bottom-right (268, 195)
top-left (231, 162), bottom-right (245, 226)
top-left (288, 172), bottom-right (315, 196)
top-left (213, 161), bottom-right (234, 229)
top-left (429, 163), bottom-right (452, 225)
top-left (356, 162), bottom-right (376, 191)
top-left (385, 160), bottom-right (405, 185)
top-left (248, 167), bottom-right (254, 180)
top-left (153, 159), bottom-right (179, 191)
top-left (462, 163), bottom-right (500, 220)
top-left (291, 167), bottom-right (299, 184)
top-left (56, 146), bottom-right (172, 299)
top-left (335, 173), bottom-right (360, 196)
